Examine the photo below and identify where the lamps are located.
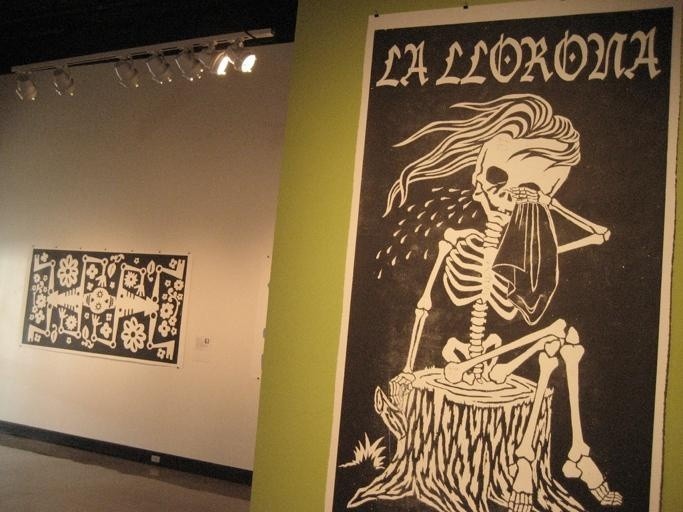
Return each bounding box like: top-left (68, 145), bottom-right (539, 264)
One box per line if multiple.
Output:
top-left (12, 26), bottom-right (276, 103)
